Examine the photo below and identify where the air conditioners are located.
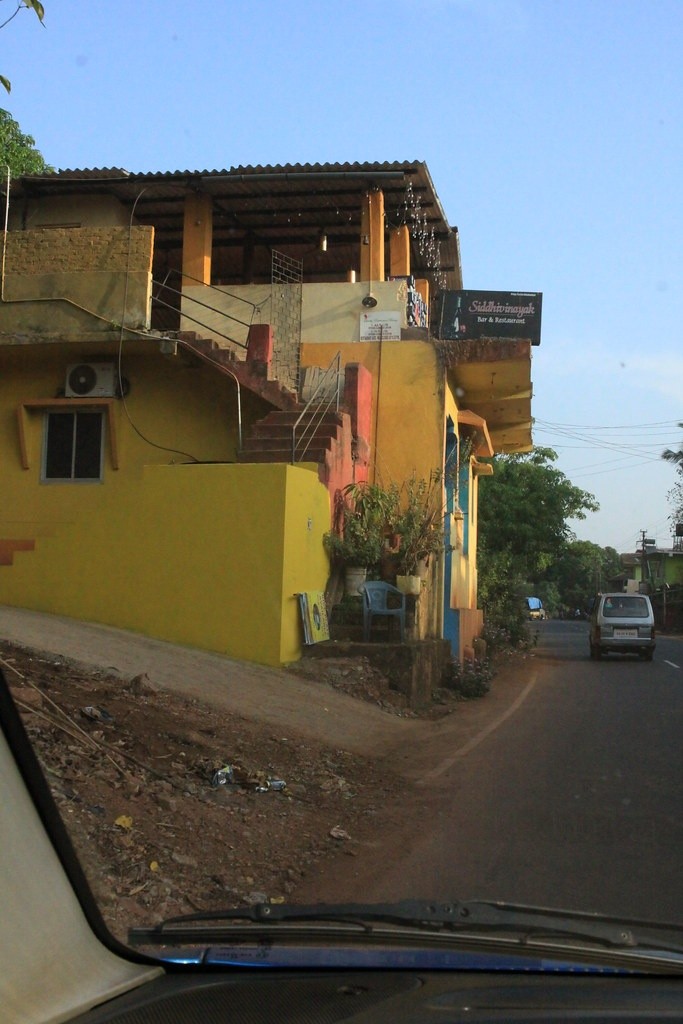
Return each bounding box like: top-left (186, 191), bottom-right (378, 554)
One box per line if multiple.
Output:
top-left (64, 360), bottom-right (119, 398)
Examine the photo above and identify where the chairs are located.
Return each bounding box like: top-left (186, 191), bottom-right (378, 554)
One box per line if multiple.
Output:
top-left (356, 582), bottom-right (406, 644)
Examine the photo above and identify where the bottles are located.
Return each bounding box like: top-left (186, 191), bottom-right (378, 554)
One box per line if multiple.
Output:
top-left (264, 780), bottom-right (287, 792)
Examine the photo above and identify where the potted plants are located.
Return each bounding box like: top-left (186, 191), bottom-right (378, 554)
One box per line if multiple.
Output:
top-left (329, 429), bottom-right (482, 596)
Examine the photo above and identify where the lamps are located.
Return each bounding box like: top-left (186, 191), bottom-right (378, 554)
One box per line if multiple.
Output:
top-left (363, 293), bottom-right (378, 308)
top-left (318, 224), bottom-right (332, 256)
top-left (455, 508), bottom-right (464, 550)
top-left (360, 231), bottom-right (370, 245)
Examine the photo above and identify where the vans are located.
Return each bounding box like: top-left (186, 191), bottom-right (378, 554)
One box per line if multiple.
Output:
top-left (584, 591), bottom-right (657, 661)
top-left (525, 595), bottom-right (545, 619)
